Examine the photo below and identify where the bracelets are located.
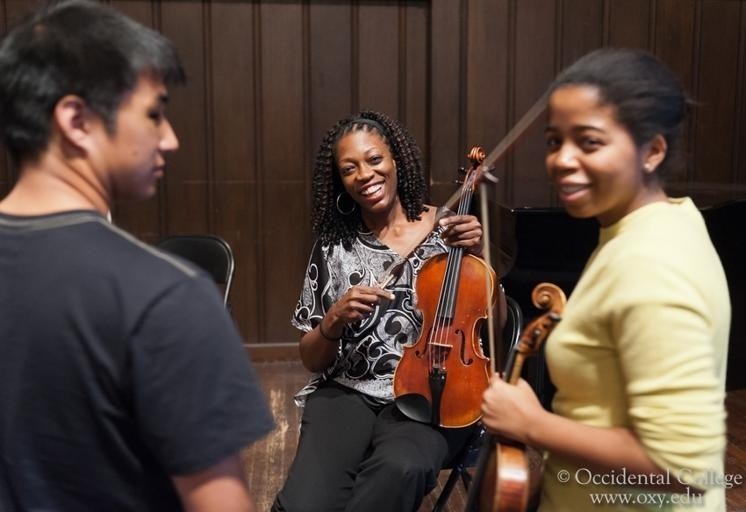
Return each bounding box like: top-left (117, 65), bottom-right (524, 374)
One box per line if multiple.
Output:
top-left (319, 320), bottom-right (344, 341)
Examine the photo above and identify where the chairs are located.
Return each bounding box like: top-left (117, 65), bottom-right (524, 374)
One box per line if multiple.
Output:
top-left (156, 234), bottom-right (236, 308)
top-left (432, 293), bottom-right (524, 511)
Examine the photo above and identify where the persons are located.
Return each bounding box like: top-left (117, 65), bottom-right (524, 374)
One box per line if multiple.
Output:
top-left (267, 108), bottom-right (489, 512)
top-left (0, 0), bottom-right (285, 512)
top-left (480, 44), bottom-right (732, 511)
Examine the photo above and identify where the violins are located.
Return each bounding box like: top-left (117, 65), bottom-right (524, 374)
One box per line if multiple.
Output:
top-left (392, 145), bottom-right (501, 428)
top-left (458, 281), bottom-right (565, 512)
